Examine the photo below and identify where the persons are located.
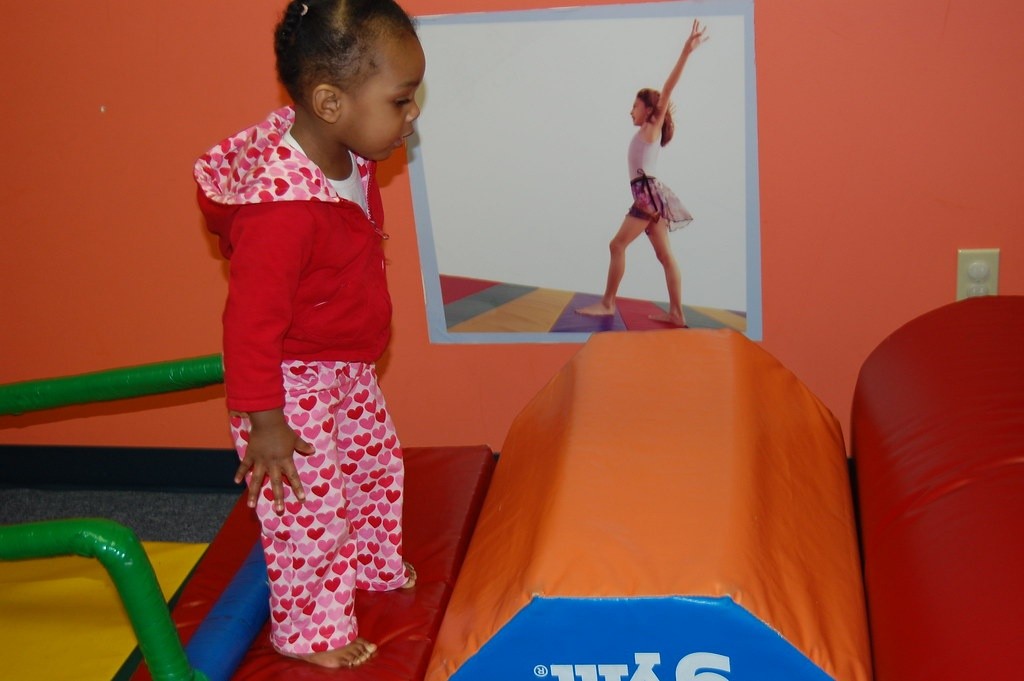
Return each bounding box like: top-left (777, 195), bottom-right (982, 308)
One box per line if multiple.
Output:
top-left (576, 19), bottom-right (709, 326)
top-left (194, 1), bottom-right (425, 668)
